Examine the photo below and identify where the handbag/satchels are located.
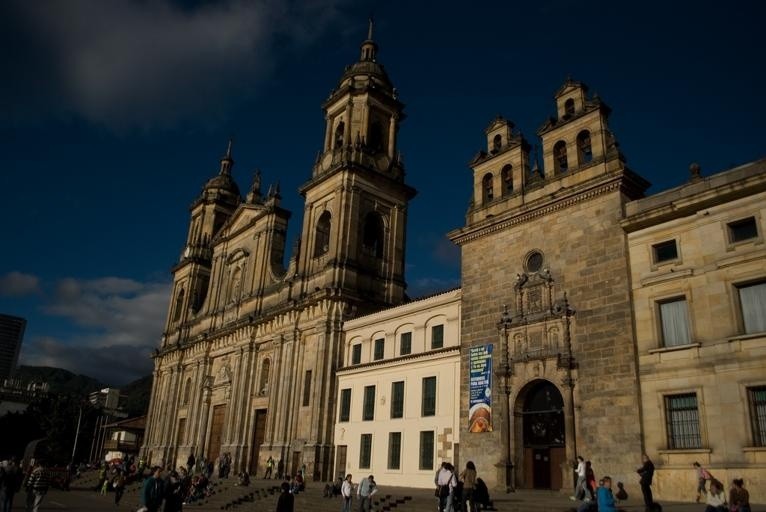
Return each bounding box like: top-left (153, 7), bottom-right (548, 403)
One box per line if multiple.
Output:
top-left (434, 484), bottom-right (451, 498)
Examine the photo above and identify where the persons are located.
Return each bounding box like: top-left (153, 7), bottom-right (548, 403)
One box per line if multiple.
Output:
top-left (726, 478), bottom-right (752, 512)
top-left (569, 456), bottom-right (629, 511)
top-left (634, 454), bottom-right (656, 512)
top-left (1, 450), bottom-right (379, 510)
top-left (703, 479), bottom-right (728, 512)
top-left (691, 461), bottom-right (707, 503)
top-left (434, 460), bottom-right (494, 511)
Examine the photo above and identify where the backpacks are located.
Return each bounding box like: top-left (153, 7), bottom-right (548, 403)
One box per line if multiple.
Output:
top-left (700, 468), bottom-right (713, 480)
